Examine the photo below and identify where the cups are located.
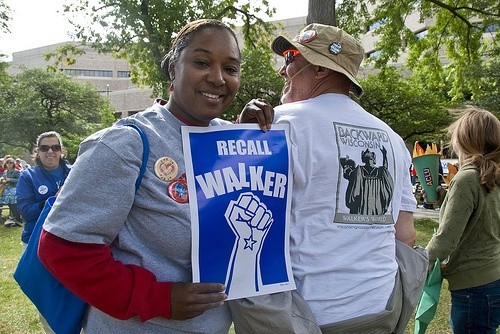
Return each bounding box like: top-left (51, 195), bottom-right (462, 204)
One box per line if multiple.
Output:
top-left (411, 152), bottom-right (442, 202)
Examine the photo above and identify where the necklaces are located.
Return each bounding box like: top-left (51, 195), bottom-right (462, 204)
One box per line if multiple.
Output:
top-left (45, 169), bottom-right (64, 190)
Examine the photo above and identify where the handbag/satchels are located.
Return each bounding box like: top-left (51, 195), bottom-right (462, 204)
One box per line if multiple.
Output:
top-left (14, 123), bottom-right (150, 334)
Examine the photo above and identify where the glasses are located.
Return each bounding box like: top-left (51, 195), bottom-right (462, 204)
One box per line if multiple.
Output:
top-left (282, 50), bottom-right (301, 64)
top-left (38, 144), bottom-right (61, 152)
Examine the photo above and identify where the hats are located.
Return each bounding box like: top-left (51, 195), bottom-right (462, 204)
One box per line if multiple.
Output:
top-left (272, 23), bottom-right (365, 100)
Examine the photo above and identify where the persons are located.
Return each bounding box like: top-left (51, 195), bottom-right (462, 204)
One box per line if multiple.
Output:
top-left (38, 18), bottom-right (274, 334)
top-left (16, 131), bottom-right (72, 254)
top-left (0, 154), bottom-right (36, 227)
top-left (420, 109), bottom-right (500, 334)
top-left (271, 22), bottom-right (429, 334)
